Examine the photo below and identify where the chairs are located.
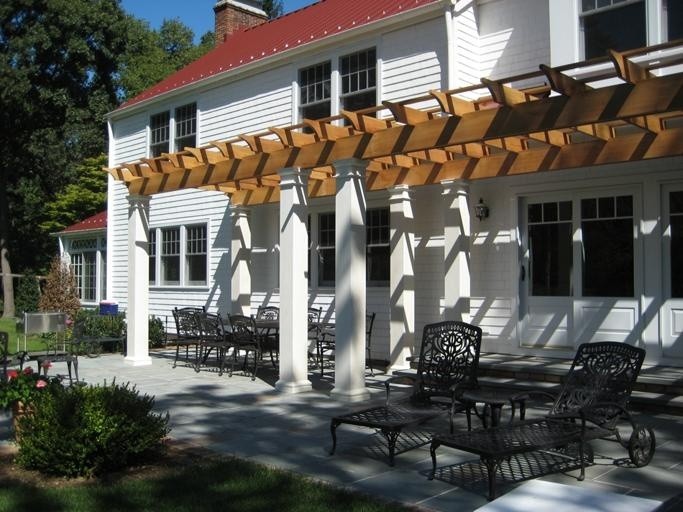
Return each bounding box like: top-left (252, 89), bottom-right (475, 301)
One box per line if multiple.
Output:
top-left (427, 340), bottom-right (656, 501)
top-left (330, 321), bottom-right (482, 467)
top-left (0, 320), bottom-right (84, 390)
top-left (171, 305), bottom-right (376, 381)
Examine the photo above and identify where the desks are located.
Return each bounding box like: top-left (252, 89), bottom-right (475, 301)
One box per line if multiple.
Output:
top-left (460, 387), bottom-right (532, 465)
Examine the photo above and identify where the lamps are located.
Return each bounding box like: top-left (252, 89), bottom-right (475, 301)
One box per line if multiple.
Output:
top-left (473, 196), bottom-right (489, 223)
